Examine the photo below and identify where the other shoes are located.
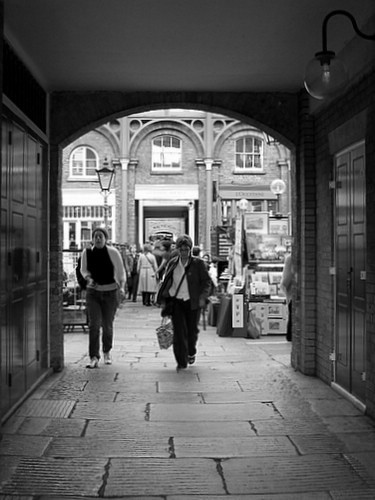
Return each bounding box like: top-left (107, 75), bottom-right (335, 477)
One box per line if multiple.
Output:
top-left (177, 366), bottom-right (188, 372)
top-left (189, 355), bottom-right (195, 364)
top-left (86, 357), bottom-right (98, 368)
top-left (103, 351), bottom-right (112, 364)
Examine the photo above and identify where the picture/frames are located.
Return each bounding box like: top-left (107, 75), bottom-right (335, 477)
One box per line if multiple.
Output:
top-left (243, 212), bottom-right (269, 234)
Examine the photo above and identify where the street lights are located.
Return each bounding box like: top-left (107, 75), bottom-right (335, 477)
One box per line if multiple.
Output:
top-left (95, 157), bottom-right (116, 245)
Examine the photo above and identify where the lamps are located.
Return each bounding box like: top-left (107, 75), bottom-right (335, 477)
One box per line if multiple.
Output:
top-left (304, 10), bottom-right (375, 100)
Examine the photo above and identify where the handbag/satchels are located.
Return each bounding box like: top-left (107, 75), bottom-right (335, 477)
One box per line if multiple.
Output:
top-left (156, 315), bottom-right (175, 349)
top-left (161, 298), bottom-right (174, 317)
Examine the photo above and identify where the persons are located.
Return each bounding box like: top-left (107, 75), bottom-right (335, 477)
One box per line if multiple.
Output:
top-left (150, 237), bottom-right (219, 306)
top-left (279, 254), bottom-right (293, 342)
top-left (79, 228), bottom-right (126, 368)
top-left (137, 243), bottom-right (159, 306)
top-left (158, 234), bottom-right (211, 370)
top-left (125, 244), bottom-right (140, 303)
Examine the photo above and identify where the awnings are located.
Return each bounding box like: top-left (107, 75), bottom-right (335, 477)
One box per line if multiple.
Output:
top-left (135, 184), bottom-right (199, 200)
top-left (218, 185), bottom-right (278, 199)
top-left (62, 188), bottom-right (116, 205)
